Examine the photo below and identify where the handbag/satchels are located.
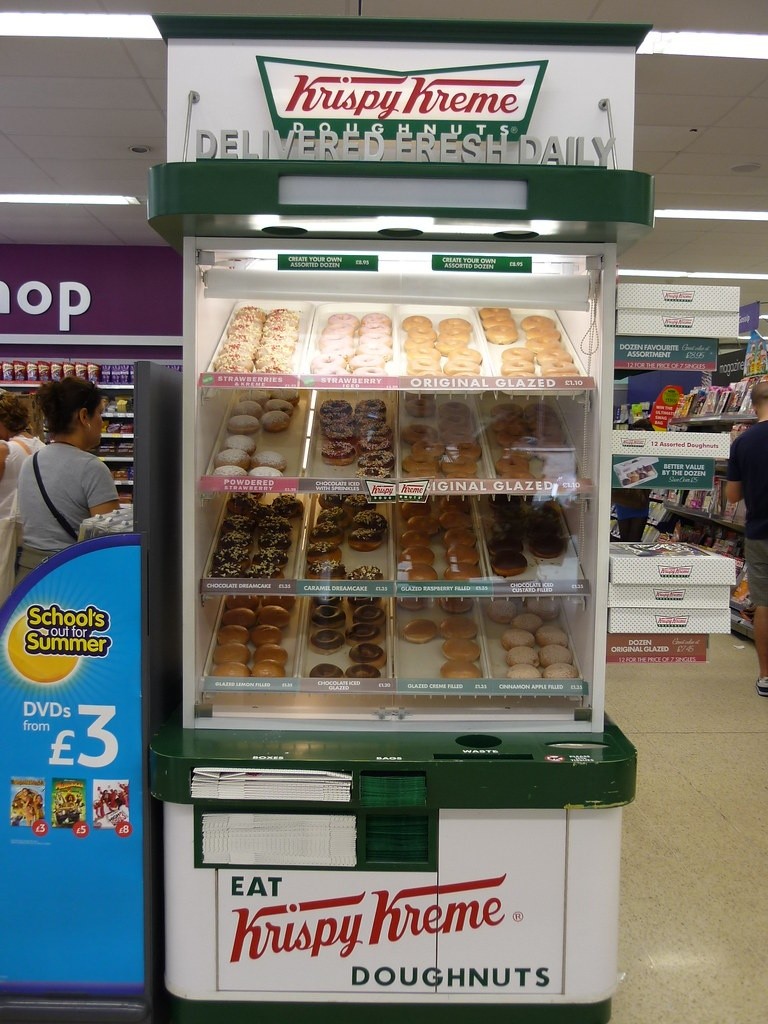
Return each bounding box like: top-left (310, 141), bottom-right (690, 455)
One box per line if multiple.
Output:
top-left (0, 485), bottom-right (23, 609)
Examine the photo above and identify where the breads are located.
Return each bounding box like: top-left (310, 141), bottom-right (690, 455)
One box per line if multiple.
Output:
top-left (400, 496), bottom-right (481, 580)
top-left (487, 598), bottom-right (579, 679)
top-left (212, 595), bottom-right (295, 678)
top-left (215, 305), bottom-right (298, 376)
top-left (212, 389), bottom-right (300, 479)
top-left (488, 494), bottom-right (567, 576)
top-left (398, 596), bottom-right (481, 677)
top-left (207, 493), bottom-right (303, 579)
top-left (306, 494), bottom-right (387, 580)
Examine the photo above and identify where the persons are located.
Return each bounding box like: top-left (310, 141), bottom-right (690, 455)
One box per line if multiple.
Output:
top-left (96, 783), bottom-right (125, 812)
top-left (615, 419), bottom-right (655, 542)
top-left (15, 376), bottom-right (120, 588)
top-left (14, 788), bottom-right (44, 826)
top-left (726, 381), bottom-right (768, 698)
top-left (0, 393), bottom-right (47, 578)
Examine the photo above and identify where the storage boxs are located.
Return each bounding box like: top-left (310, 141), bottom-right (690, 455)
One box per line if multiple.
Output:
top-left (607, 540), bottom-right (736, 634)
top-left (616, 283), bottom-right (740, 338)
top-left (612, 430), bottom-right (731, 460)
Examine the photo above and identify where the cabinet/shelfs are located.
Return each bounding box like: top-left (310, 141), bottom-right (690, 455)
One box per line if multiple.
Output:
top-left (606, 335), bottom-right (719, 661)
top-left (179, 236), bottom-right (618, 733)
top-left (0, 379), bottom-right (134, 487)
top-left (656, 414), bottom-right (758, 641)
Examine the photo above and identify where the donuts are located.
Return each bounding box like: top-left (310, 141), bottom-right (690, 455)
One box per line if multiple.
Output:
top-left (310, 307), bottom-right (579, 378)
top-left (622, 465), bottom-right (653, 485)
top-left (309, 596), bottom-right (386, 678)
top-left (320, 397), bottom-right (567, 479)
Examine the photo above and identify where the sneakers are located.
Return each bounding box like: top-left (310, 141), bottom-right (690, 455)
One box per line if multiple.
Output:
top-left (755, 675), bottom-right (768, 697)
